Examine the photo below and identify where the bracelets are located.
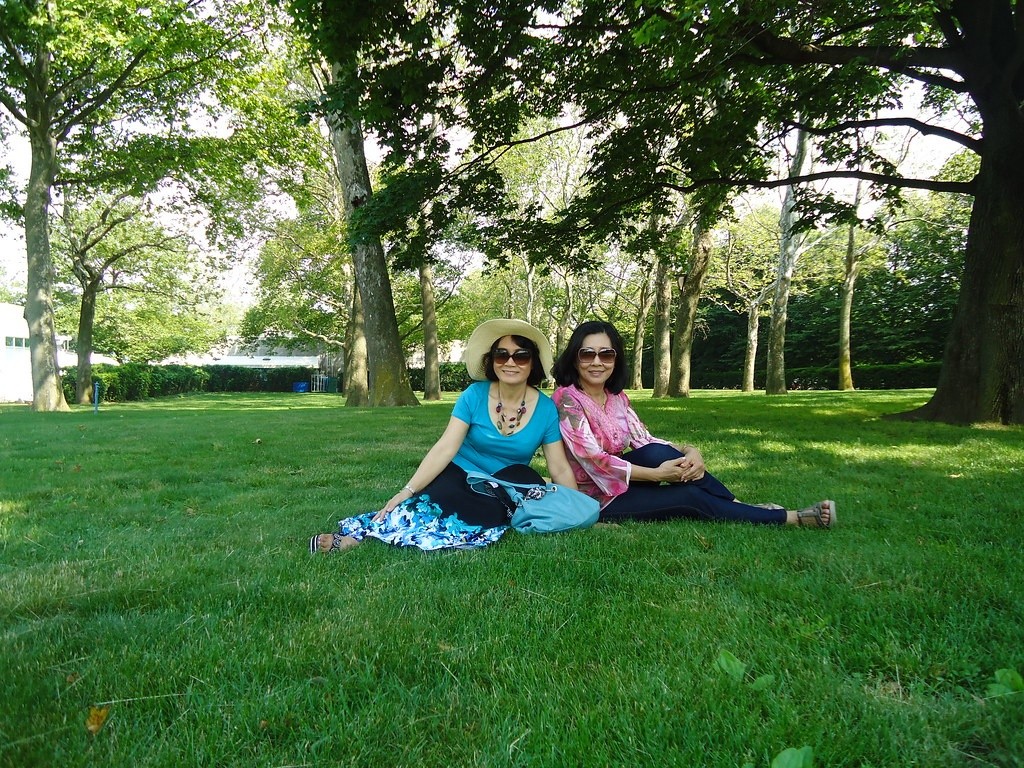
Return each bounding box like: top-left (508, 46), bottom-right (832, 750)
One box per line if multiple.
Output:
top-left (404, 485), bottom-right (415, 494)
top-left (681, 444), bottom-right (694, 452)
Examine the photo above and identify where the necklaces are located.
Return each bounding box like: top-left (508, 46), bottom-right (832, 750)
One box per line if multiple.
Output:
top-left (496, 381), bottom-right (527, 435)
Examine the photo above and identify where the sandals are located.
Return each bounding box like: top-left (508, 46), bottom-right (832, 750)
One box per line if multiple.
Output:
top-left (748, 502), bottom-right (784, 510)
top-left (309, 534), bottom-right (360, 556)
top-left (797, 500), bottom-right (836, 529)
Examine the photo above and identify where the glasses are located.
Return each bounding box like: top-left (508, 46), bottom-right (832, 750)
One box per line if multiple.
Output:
top-left (492, 348), bottom-right (531, 365)
top-left (578, 348), bottom-right (618, 363)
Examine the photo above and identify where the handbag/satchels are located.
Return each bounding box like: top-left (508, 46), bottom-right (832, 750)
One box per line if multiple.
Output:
top-left (466, 464), bottom-right (601, 538)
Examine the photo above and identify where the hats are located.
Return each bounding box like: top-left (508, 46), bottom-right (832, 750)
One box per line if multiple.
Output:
top-left (465, 319), bottom-right (552, 382)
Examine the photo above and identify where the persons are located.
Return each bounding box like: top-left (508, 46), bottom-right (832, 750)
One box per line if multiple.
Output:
top-left (310, 323), bottom-right (578, 556)
top-left (551, 320), bottom-right (836, 529)
